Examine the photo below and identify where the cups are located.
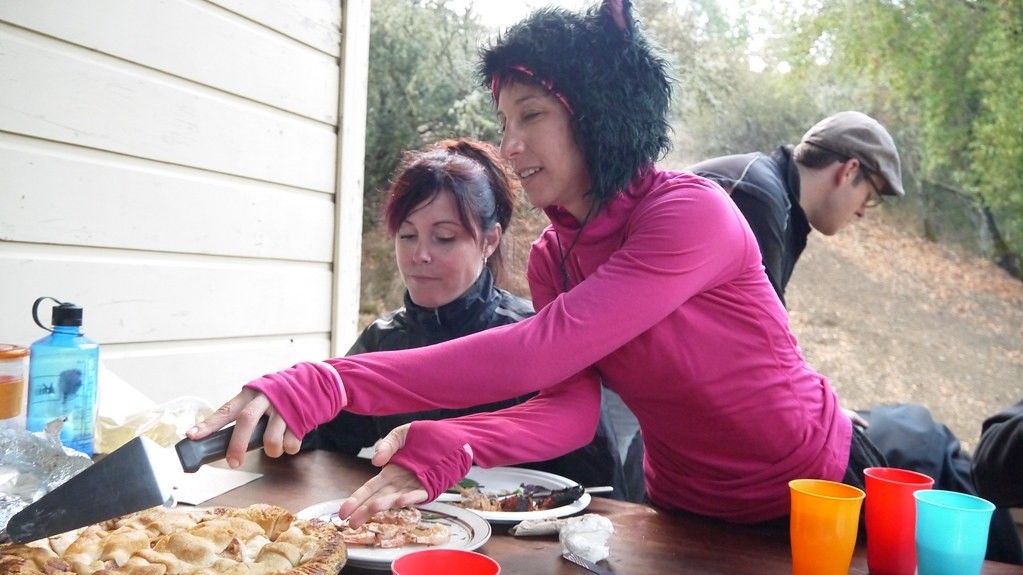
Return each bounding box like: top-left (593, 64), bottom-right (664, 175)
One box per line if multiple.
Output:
top-left (788, 478), bottom-right (866, 575)
top-left (863, 466), bottom-right (935, 575)
top-left (912, 489), bottom-right (997, 575)
top-left (390, 548), bottom-right (501, 575)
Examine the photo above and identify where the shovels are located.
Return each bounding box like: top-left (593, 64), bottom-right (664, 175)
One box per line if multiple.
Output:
top-left (7, 414), bottom-right (270, 545)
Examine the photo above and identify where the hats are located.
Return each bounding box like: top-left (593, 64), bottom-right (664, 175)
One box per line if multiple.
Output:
top-left (802, 111), bottom-right (906, 197)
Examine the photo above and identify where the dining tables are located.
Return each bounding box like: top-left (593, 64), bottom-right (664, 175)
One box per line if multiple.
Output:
top-left (176, 450), bottom-right (1022, 575)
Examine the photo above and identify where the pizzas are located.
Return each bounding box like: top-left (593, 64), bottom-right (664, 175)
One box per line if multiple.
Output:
top-left (0, 503), bottom-right (348, 575)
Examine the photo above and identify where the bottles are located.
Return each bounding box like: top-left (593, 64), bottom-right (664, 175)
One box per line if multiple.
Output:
top-left (26, 295), bottom-right (99, 458)
top-left (0, 341), bottom-right (32, 474)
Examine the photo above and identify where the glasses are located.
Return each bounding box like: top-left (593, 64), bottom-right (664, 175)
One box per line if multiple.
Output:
top-left (838, 158), bottom-right (885, 208)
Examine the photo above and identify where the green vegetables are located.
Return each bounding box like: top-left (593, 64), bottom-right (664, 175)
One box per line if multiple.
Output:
top-left (457, 478), bottom-right (484, 488)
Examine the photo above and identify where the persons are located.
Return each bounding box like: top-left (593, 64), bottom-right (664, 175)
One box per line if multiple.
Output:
top-left (299, 140), bottom-right (626, 503)
top-left (599, 110), bottom-right (905, 503)
top-left (186, 8), bottom-right (889, 548)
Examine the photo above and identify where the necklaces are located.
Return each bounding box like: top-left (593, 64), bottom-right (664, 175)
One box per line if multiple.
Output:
top-left (557, 202), bottom-right (594, 293)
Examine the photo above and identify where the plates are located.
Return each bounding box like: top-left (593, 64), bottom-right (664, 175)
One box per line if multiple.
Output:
top-left (433, 465), bottom-right (592, 524)
top-left (289, 496), bottom-right (492, 571)
